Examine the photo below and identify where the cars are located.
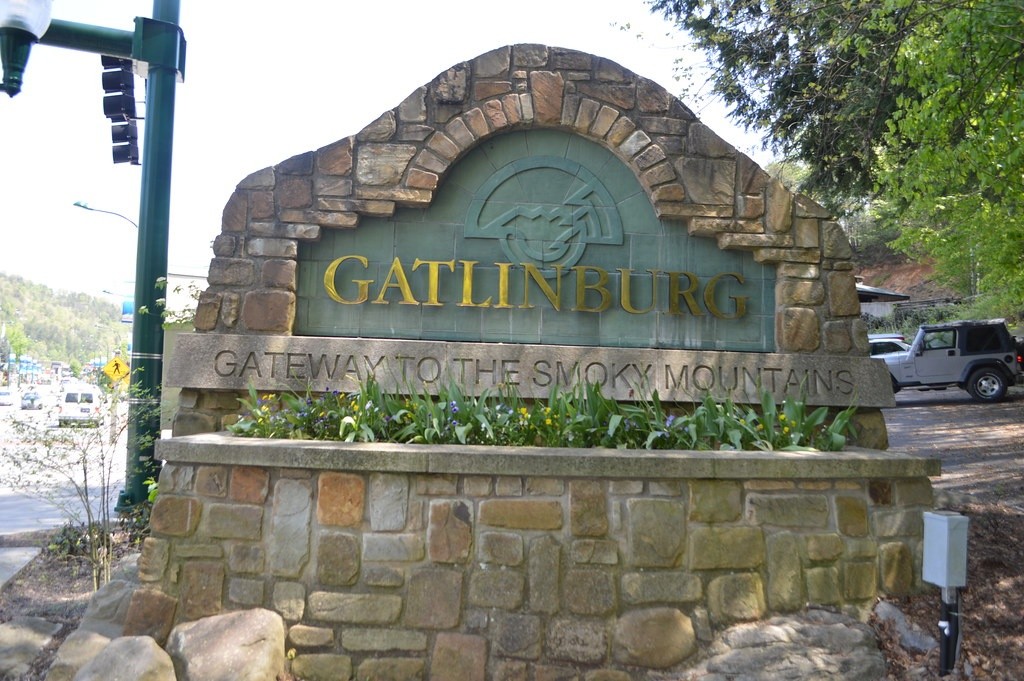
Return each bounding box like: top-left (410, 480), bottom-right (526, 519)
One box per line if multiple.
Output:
top-left (0, 386), bottom-right (13, 408)
top-left (21, 394), bottom-right (44, 410)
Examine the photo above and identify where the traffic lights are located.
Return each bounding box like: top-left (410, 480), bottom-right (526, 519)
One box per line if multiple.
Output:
top-left (101, 54), bottom-right (139, 166)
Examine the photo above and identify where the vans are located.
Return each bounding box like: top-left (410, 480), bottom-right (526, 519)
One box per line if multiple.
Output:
top-left (58, 386), bottom-right (104, 427)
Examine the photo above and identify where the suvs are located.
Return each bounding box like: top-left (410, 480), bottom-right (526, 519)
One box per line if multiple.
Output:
top-left (869, 318), bottom-right (1024, 403)
top-left (867, 333), bottom-right (912, 355)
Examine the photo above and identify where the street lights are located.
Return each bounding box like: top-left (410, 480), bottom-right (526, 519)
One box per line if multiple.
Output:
top-left (0, 0), bottom-right (187, 520)
